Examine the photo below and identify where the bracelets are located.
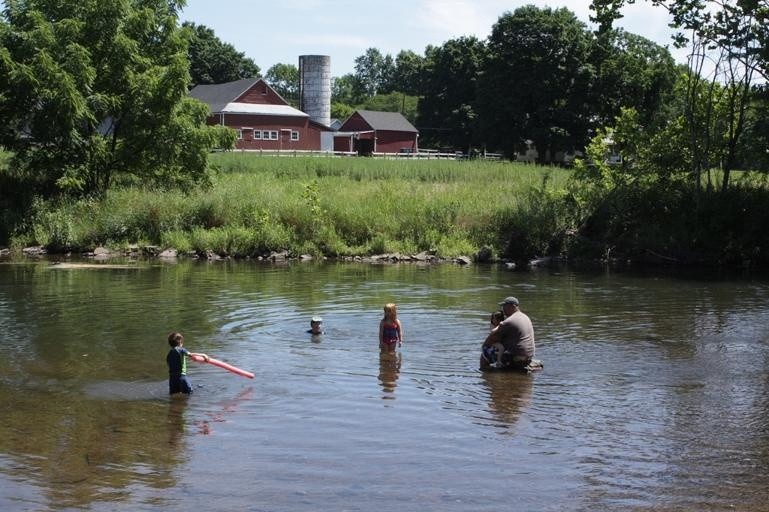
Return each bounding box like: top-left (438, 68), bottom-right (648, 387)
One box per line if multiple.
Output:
top-left (399, 340), bottom-right (402, 341)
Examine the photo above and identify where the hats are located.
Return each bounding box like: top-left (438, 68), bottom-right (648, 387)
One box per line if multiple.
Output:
top-left (498, 296), bottom-right (520, 307)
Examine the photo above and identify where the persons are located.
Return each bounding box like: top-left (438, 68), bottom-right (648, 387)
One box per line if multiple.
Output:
top-left (479, 295), bottom-right (536, 374)
top-left (165, 333), bottom-right (210, 396)
top-left (378, 301), bottom-right (402, 351)
top-left (489, 309), bottom-right (510, 368)
top-left (305, 316), bottom-right (326, 335)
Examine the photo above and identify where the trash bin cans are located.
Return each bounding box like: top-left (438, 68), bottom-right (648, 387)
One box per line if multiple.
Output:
top-left (401, 148), bottom-right (412, 157)
